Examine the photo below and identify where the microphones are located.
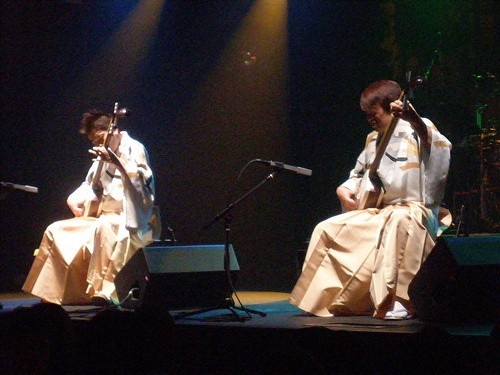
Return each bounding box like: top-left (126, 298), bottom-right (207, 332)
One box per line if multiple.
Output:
top-left (256, 159), bottom-right (312, 176)
top-left (0, 182), bottom-right (38, 193)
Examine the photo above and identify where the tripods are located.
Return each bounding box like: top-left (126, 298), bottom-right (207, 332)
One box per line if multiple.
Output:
top-left (172, 170), bottom-right (279, 323)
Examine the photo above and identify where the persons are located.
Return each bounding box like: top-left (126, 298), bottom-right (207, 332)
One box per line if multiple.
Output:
top-left (23, 111), bottom-right (161, 305)
top-left (287, 81), bottom-right (453, 320)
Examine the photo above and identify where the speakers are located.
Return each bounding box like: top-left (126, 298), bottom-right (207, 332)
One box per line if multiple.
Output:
top-left (408, 235), bottom-right (500, 328)
top-left (114, 244), bottom-right (240, 311)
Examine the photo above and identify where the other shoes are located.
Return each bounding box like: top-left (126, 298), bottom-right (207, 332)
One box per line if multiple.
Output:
top-left (384, 310), bottom-right (415, 320)
top-left (91, 291), bottom-right (113, 307)
